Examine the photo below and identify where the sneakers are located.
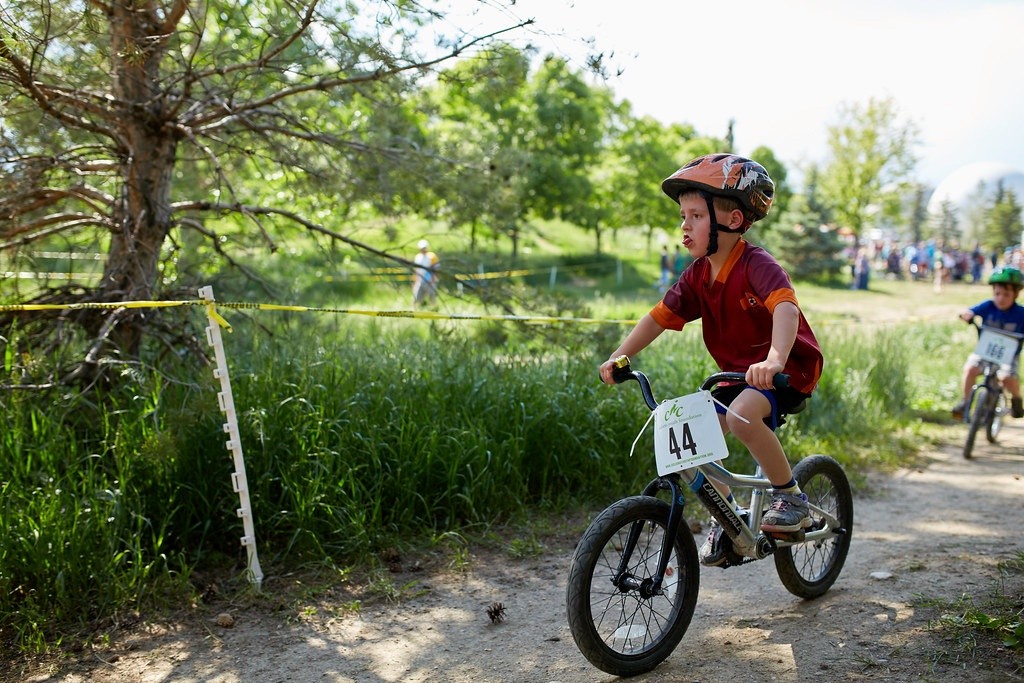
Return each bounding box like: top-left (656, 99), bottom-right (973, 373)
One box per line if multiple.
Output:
top-left (698, 506), bottom-right (747, 566)
top-left (761, 491), bottom-right (813, 532)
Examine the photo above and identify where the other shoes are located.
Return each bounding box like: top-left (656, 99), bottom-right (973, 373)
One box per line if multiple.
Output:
top-left (951, 404), bottom-right (970, 414)
top-left (1011, 399), bottom-right (1024, 418)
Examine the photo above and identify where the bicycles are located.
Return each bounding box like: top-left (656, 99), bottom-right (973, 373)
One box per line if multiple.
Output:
top-left (952, 314), bottom-right (1023, 459)
top-left (566, 353), bottom-right (853, 680)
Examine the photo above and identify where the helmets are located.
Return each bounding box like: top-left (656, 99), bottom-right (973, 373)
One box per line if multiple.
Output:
top-left (662, 153), bottom-right (775, 222)
top-left (988, 267), bottom-right (1024, 290)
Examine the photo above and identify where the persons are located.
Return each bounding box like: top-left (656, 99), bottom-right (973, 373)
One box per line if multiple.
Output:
top-left (657, 232), bottom-right (1023, 298)
top-left (951, 264), bottom-right (1024, 420)
top-left (412, 239), bottom-right (440, 310)
top-left (598, 152), bottom-right (824, 567)
